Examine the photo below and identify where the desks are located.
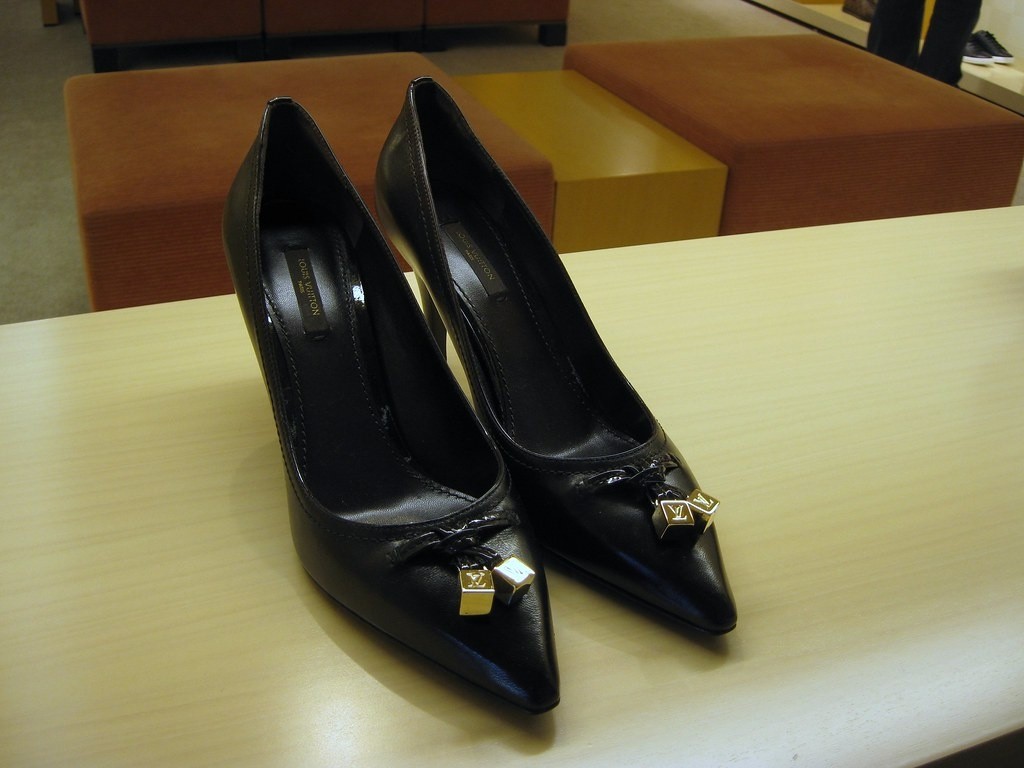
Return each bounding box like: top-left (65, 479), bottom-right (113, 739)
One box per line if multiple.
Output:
top-left (0, 204), bottom-right (1024, 768)
top-left (449, 71), bottom-right (731, 258)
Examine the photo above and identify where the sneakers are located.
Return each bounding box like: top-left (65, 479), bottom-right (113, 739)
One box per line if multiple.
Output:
top-left (973, 30), bottom-right (1015, 64)
top-left (961, 33), bottom-right (995, 65)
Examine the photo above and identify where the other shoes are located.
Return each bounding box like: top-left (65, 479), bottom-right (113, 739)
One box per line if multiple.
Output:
top-left (842, 0), bottom-right (877, 22)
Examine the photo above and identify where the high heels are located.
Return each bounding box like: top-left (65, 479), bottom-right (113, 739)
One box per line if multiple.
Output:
top-left (375, 78), bottom-right (738, 638)
top-left (222, 95), bottom-right (559, 713)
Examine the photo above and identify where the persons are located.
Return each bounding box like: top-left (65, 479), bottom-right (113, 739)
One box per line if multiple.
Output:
top-left (866, 0), bottom-right (982, 87)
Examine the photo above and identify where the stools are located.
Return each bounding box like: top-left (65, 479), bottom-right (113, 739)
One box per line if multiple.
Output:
top-left (63, 48), bottom-right (557, 312)
top-left (564, 33), bottom-right (1024, 237)
